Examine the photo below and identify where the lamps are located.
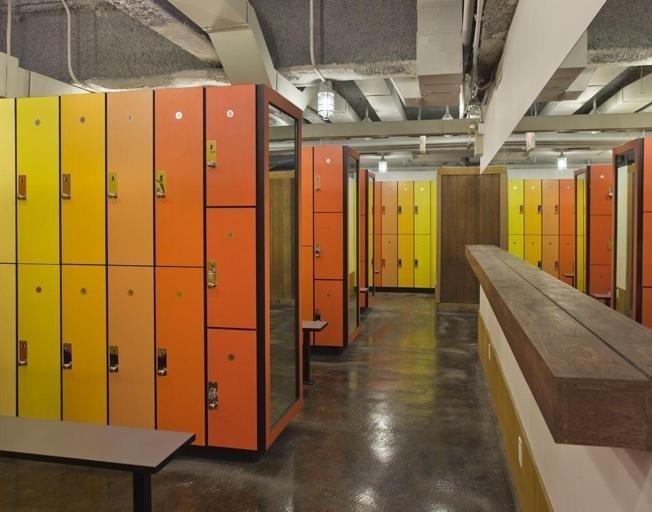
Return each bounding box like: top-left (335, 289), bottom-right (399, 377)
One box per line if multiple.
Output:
top-left (556, 149), bottom-right (568, 172)
top-left (379, 154), bottom-right (388, 173)
top-left (317, 80), bottom-right (335, 120)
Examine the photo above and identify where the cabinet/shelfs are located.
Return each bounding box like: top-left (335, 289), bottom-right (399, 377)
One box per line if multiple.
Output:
top-left (301, 144), bottom-right (376, 354)
top-left (0, 83), bottom-right (305, 459)
top-left (373, 178), bottom-right (575, 289)
top-left (573, 136), bottom-right (652, 330)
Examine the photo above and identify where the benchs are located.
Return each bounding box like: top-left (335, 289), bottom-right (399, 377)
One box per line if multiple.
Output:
top-left (300, 320), bottom-right (328, 385)
top-left (1, 414), bottom-right (196, 512)
